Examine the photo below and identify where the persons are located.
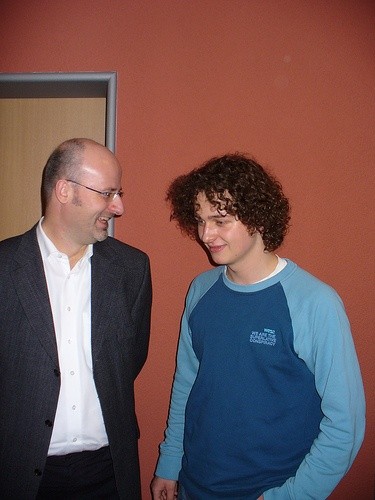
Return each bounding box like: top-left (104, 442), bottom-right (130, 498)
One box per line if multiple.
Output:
top-left (149, 153), bottom-right (367, 500)
top-left (0, 136), bottom-right (154, 500)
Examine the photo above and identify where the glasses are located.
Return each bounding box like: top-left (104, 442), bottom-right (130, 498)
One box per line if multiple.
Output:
top-left (66, 178), bottom-right (124, 206)
